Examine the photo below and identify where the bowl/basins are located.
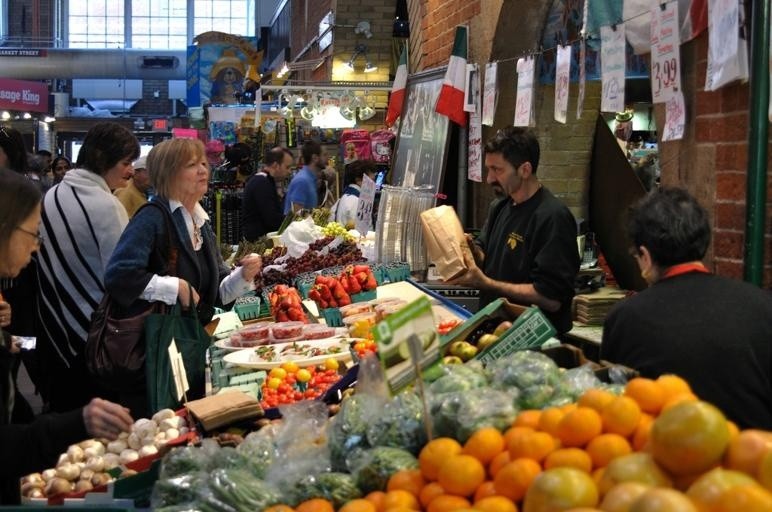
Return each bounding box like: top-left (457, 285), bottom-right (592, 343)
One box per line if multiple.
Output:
top-left (229, 321), bottom-right (335, 347)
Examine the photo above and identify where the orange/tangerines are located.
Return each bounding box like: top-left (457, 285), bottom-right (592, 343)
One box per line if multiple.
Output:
top-left (262, 374), bottom-right (772, 512)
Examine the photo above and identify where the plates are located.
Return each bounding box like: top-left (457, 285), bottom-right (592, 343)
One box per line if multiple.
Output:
top-left (213, 327), bottom-right (349, 352)
top-left (221, 338), bottom-right (366, 371)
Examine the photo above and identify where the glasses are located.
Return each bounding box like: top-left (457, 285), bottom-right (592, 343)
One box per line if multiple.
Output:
top-left (14, 224), bottom-right (44, 248)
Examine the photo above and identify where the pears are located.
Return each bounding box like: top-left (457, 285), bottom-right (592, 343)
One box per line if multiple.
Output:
top-left (442, 320), bottom-right (512, 367)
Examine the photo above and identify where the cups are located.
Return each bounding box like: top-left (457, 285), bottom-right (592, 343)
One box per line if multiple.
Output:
top-left (339, 296), bottom-right (409, 339)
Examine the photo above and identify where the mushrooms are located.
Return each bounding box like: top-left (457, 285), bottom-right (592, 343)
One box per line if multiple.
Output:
top-left (20, 408), bottom-right (190, 500)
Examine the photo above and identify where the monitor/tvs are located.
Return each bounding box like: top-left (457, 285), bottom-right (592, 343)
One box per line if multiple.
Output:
top-left (70, 139), bottom-right (84, 164)
top-left (131, 139), bottom-right (155, 169)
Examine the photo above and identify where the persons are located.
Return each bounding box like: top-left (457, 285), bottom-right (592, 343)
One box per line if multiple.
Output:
top-left (599, 185), bottom-right (772, 430)
top-left (0, 121), bottom-right (261, 512)
top-left (439, 126), bottom-right (578, 341)
top-left (241, 140), bottom-right (377, 250)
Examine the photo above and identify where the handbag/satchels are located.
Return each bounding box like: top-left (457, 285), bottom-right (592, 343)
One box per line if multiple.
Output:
top-left (420, 204), bottom-right (467, 283)
top-left (84, 292), bottom-right (212, 417)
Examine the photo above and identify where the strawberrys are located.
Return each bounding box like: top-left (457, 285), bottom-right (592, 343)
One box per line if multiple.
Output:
top-left (267, 263), bottom-right (377, 323)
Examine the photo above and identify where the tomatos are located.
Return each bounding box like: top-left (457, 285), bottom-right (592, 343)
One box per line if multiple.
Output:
top-left (260, 358), bottom-right (339, 381)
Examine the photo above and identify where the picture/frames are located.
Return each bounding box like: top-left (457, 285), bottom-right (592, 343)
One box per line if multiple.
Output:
top-left (386, 64), bottom-right (455, 208)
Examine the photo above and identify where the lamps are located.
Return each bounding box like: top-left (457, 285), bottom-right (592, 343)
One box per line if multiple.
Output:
top-left (354, 21), bottom-right (375, 41)
top-left (275, 56), bottom-right (327, 80)
top-left (342, 42), bottom-right (378, 74)
top-left (275, 88), bottom-right (377, 123)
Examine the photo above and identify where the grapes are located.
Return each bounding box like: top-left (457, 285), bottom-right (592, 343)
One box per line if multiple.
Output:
top-left (232, 221), bottom-right (367, 290)
